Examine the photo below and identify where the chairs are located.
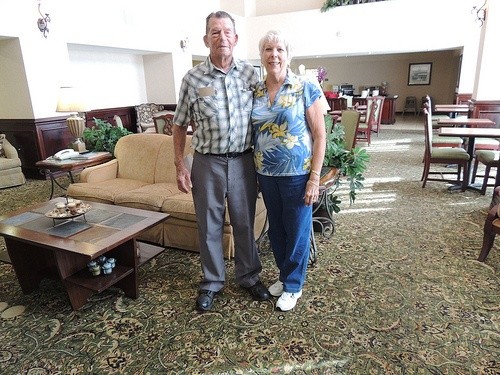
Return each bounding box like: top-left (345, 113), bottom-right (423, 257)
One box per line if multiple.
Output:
top-left (134, 103), bottom-right (165, 133)
top-left (355, 101), bottom-right (378, 145)
top-left (359, 95), bottom-right (387, 137)
top-left (152, 114), bottom-right (175, 136)
top-left (327, 94), bottom-right (354, 112)
top-left (338, 108), bottom-right (362, 178)
top-left (324, 115), bottom-right (339, 141)
top-left (113, 114), bottom-right (125, 132)
top-left (403, 96), bottom-right (418, 119)
top-left (153, 110), bottom-right (177, 117)
top-left (420, 95), bottom-right (500, 262)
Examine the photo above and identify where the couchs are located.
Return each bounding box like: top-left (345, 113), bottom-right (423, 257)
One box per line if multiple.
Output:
top-left (64, 133), bottom-right (268, 260)
top-left (0, 134), bottom-right (27, 189)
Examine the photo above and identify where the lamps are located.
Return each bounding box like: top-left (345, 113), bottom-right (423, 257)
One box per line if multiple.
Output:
top-left (55, 84), bottom-right (92, 153)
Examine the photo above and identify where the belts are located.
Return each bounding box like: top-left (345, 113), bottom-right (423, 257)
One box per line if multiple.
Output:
top-left (206, 148), bottom-right (252, 158)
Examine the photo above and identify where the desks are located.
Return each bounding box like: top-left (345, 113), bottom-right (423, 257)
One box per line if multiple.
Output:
top-left (434, 108), bottom-right (470, 128)
top-left (327, 95), bottom-right (399, 124)
top-left (437, 118), bottom-right (497, 170)
top-left (434, 104), bottom-right (469, 119)
top-left (438, 126), bottom-right (500, 195)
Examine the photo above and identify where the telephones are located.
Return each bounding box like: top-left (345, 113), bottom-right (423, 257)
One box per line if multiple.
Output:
top-left (55, 148), bottom-right (80, 160)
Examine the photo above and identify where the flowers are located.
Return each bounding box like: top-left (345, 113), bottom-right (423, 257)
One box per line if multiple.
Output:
top-left (315, 65), bottom-right (329, 86)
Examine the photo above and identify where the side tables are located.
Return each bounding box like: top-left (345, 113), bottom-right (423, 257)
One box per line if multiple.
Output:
top-left (35, 149), bottom-right (113, 200)
top-left (256, 164), bottom-right (342, 268)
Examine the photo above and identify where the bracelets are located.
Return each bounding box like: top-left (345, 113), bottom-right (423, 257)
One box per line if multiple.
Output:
top-left (311, 170), bottom-right (320, 177)
top-left (307, 180), bottom-right (320, 187)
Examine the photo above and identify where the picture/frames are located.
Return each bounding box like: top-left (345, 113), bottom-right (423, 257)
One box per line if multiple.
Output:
top-left (407, 62), bottom-right (433, 87)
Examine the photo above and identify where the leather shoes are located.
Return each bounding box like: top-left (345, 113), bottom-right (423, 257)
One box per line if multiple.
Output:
top-left (240, 281), bottom-right (270, 300)
top-left (197, 290), bottom-right (220, 310)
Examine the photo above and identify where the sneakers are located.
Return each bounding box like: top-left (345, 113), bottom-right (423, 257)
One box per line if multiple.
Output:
top-left (276, 289), bottom-right (302, 311)
top-left (268, 280), bottom-right (284, 296)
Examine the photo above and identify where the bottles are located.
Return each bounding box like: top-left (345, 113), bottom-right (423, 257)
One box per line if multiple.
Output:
top-left (102, 262), bottom-right (112, 274)
top-left (87, 260), bottom-right (98, 272)
top-left (106, 258), bottom-right (116, 268)
top-left (90, 264), bottom-right (101, 275)
top-left (97, 254), bottom-right (107, 265)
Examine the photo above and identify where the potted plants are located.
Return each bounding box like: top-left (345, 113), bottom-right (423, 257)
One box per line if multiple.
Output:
top-left (311, 114), bottom-right (371, 233)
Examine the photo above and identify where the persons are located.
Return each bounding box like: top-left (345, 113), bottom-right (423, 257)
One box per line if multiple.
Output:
top-left (246, 30), bottom-right (330, 313)
top-left (171, 9), bottom-right (271, 309)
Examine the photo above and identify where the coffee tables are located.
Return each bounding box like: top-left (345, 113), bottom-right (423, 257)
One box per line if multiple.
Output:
top-left (0, 197), bottom-right (171, 311)
